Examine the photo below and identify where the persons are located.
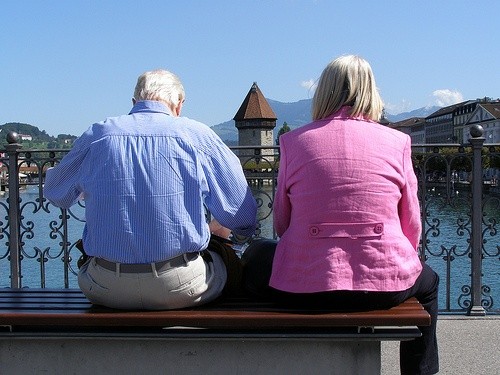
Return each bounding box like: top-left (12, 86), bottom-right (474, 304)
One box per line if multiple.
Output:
top-left (241, 54), bottom-right (440, 375)
top-left (43, 70), bottom-right (259, 313)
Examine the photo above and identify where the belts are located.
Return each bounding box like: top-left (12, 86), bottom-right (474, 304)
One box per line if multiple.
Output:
top-left (95, 251), bottom-right (200, 274)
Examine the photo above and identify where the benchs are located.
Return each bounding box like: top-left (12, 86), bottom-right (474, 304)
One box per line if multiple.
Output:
top-left (1, 287), bottom-right (432, 374)
